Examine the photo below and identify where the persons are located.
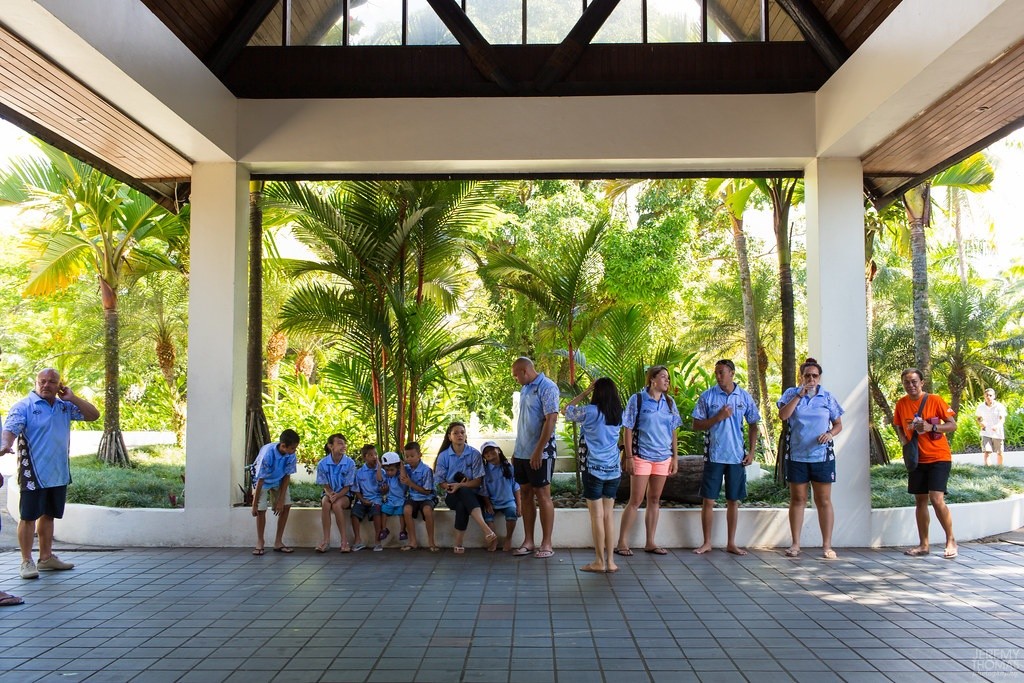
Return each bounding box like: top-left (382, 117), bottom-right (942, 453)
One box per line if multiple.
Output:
top-left (614, 365), bottom-right (683, 555)
top-left (776, 358), bottom-right (844, 560)
top-left (433, 422), bottom-right (498, 554)
top-left (561, 378), bottom-right (624, 573)
top-left (0, 348), bottom-right (25, 605)
top-left (315, 433), bottom-right (356, 551)
top-left (690, 359), bottom-right (761, 556)
top-left (349, 445), bottom-right (386, 552)
top-left (0, 368), bottom-right (101, 578)
top-left (252, 429), bottom-right (300, 555)
top-left (399, 442), bottom-right (439, 553)
top-left (481, 440), bottom-right (521, 551)
top-left (375, 452), bottom-right (408, 539)
top-left (894, 368), bottom-right (957, 558)
top-left (976, 388), bottom-right (1006, 465)
top-left (510, 357), bottom-right (560, 557)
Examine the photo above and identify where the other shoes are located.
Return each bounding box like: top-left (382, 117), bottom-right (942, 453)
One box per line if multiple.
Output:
top-left (380, 528), bottom-right (390, 540)
top-left (340, 543), bottom-right (351, 552)
top-left (20, 560), bottom-right (39, 578)
top-left (38, 555), bottom-right (75, 572)
top-left (352, 542), bottom-right (365, 551)
top-left (373, 544), bottom-right (383, 552)
top-left (400, 531), bottom-right (408, 540)
top-left (315, 543), bottom-right (329, 552)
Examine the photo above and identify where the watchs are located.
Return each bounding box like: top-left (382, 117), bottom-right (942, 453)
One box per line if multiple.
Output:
top-left (932, 424), bottom-right (937, 432)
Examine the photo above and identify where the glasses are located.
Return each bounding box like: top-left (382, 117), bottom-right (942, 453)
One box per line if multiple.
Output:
top-left (717, 360), bottom-right (733, 370)
top-left (803, 373), bottom-right (820, 378)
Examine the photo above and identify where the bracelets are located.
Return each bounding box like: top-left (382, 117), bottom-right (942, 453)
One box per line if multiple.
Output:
top-left (626, 456), bottom-right (632, 458)
top-left (829, 431), bottom-right (834, 436)
top-left (797, 394), bottom-right (802, 398)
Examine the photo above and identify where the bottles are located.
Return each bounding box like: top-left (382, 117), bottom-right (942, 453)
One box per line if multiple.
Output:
top-left (913, 413), bottom-right (926, 434)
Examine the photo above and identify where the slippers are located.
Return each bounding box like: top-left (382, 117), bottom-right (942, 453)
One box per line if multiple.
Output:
top-left (0, 595), bottom-right (25, 605)
top-left (580, 564), bottom-right (606, 573)
top-left (614, 548), bottom-right (633, 556)
top-left (454, 546), bottom-right (464, 554)
top-left (485, 533), bottom-right (497, 542)
top-left (534, 550), bottom-right (555, 558)
top-left (273, 545), bottom-right (294, 553)
top-left (429, 545), bottom-right (439, 553)
top-left (605, 567), bottom-right (615, 573)
top-left (513, 546), bottom-right (534, 556)
top-left (401, 544), bottom-right (417, 551)
top-left (944, 541), bottom-right (958, 558)
top-left (253, 549), bottom-right (264, 555)
top-left (644, 546), bottom-right (667, 555)
top-left (905, 546), bottom-right (929, 556)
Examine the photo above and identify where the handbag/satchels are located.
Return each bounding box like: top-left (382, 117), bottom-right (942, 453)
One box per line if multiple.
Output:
top-left (903, 440), bottom-right (917, 471)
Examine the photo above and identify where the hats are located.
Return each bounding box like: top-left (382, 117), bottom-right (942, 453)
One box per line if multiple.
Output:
top-left (480, 441), bottom-right (498, 453)
top-left (381, 452), bottom-right (401, 465)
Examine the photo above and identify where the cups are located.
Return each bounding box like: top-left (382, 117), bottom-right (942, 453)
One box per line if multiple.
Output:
top-left (808, 386), bottom-right (815, 396)
